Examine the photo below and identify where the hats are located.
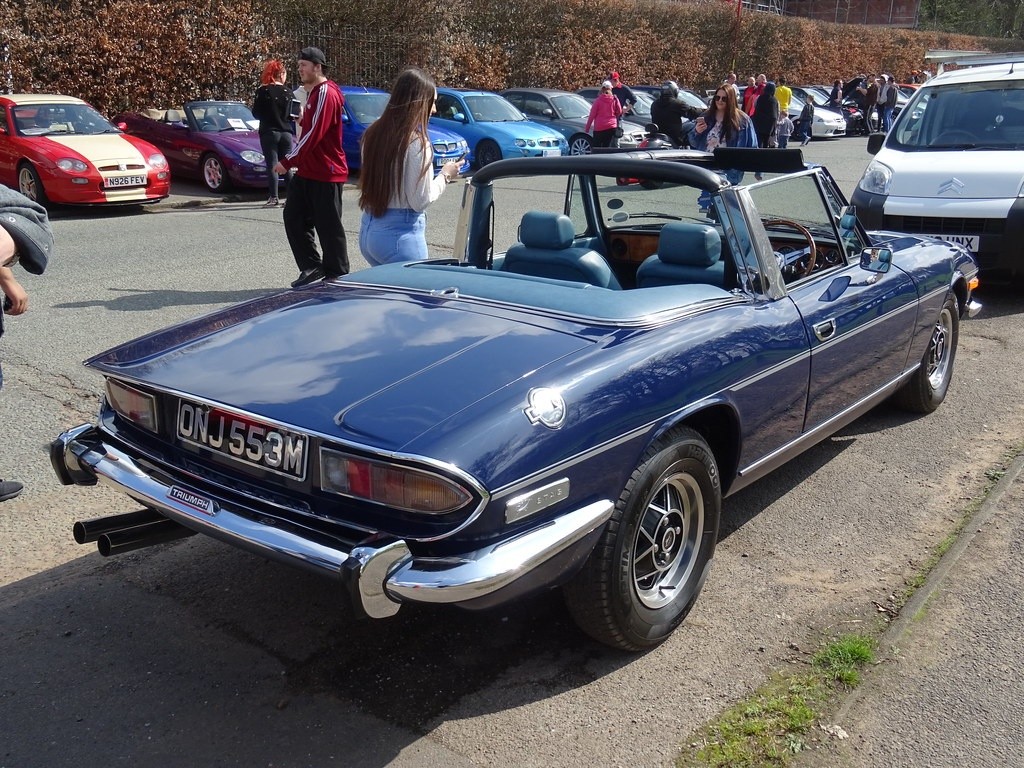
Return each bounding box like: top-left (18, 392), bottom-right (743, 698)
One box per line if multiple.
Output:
top-left (601, 80), bottom-right (614, 89)
top-left (297, 47), bottom-right (333, 68)
top-left (606, 71), bottom-right (620, 81)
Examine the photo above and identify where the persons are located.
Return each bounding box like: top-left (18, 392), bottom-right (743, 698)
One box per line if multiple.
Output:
top-left (272, 47), bottom-right (350, 288)
top-left (798, 94), bottom-right (814, 145)
top-left (691, 84), bottom-right (765, 223)
top-left (651, 81), bottom-right (704, 150)
top-left (856, 74), bottom-right (898, 136)
top-left (606, 72), bottom-right (637, 148)
top-left (829, 79), bottom-right (843, 116)
top-left (0, 182), bottom-right (55, 389)
top-left (359, 69), bottom-right (465, 267)
top-left (741, 74), bottom-right (794, 149)
top-left (252, 61), bottom-right (294, 205)
top-left (905, 69), bottom-right (931, 85)
top-left (586, 80), bottom-right (623, 148)
top-left (722, 71), bottom-right (740, 102)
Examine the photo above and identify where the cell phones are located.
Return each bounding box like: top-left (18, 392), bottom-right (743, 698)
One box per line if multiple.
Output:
top-left (697, 117), bottom-right (705, 124)
top-left (290, 99), bottom-right (301, 117)
top-left (457, 151), bottom-right (469, 162)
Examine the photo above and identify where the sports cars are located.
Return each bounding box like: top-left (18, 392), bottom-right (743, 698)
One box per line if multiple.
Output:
top-left (113, 100), bottom-right (298, 193)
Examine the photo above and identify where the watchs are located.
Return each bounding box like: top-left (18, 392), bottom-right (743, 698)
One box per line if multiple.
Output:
top-left (437, 171), bottom-right (451, 183)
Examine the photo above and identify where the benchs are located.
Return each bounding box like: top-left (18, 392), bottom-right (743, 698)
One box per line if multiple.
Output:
top-left (143, 110), bottom-right (206, 119)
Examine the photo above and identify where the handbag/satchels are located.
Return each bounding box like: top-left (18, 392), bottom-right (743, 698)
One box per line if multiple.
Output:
top-left (614, 127), bottom-right (623, 138)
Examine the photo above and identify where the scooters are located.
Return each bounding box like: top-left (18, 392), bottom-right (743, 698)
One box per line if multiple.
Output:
top-left (639, 100), bottom-right (714, 188)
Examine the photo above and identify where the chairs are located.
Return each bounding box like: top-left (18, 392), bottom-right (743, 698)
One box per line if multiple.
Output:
top-left (165, 110), bottom-right (181, 121)
top-left (500, 212), bottom-right (622, 291)
top-left (204, 107), bottom-right (218, 119)
top-left (635, 221), bottom-right (725, 287)
top-left (958, 95), bottom-right (1004, 143)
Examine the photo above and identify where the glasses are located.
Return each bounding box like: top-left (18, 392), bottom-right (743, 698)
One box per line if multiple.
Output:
top-left (713, 95), bottom-right (729, 102)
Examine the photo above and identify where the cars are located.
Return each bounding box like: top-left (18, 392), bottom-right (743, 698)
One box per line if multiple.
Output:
top-left (291, 86), bottom-right (471, 176)
top-left (0, 94), bottom-right (171, 213)
top-left (429, 87), bottom-right (572, 172)
top-left (49, 146), bottom-right (985, 649)
top-left (499, 87), bottom-right (651, 156)
top-left (848, 61), bottom-right (1024, 294)
top-left (575, 81), bottom-right (928, 138)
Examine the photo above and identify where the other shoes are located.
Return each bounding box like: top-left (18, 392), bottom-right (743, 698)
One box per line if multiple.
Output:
top-left (800, 136), bottom-right (811, 146)
top-left (291, 265), bottom-right (323, 288)
top-left (0, 480), bottom-right (24, 502)
top-left (264, 196), bottom-right (280, 209)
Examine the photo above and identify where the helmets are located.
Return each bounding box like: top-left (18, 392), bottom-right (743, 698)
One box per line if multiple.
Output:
top-left (661, 81), bottom-right (679, 98)
